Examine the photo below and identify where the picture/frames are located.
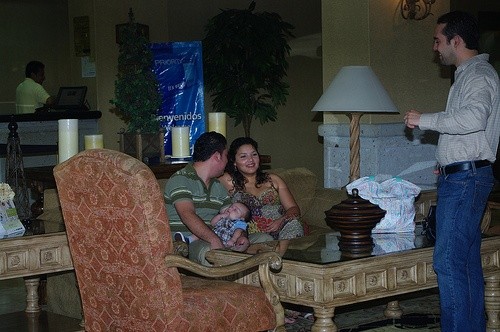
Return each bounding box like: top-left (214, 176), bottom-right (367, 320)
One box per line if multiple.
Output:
top-left (56, 86), bottom-right (88, 108)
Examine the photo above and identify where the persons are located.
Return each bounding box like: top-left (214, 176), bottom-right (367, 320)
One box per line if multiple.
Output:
top-left (15, 60), bottom-right (55, 113)
top-left (224, 135), bottom-right (316, 321)
top-left (173, 202), bottom-right (253, 248)
top-left (163, 131), bottom-right (295, 323)
top-left (404, 13), bottom-right (500, 332)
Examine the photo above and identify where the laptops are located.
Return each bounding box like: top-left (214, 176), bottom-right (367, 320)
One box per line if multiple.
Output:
top-left (51, 85), bottom-right (88, 109)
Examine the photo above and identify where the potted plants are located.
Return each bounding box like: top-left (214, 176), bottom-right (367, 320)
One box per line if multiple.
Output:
top-left (107, 7), bottom-right (164, 164)
top-left (202, 0), bottom-right (297, 175)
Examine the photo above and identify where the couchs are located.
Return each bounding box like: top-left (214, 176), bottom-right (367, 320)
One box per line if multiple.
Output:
top-left (0, 172), bottom-right (348, 320)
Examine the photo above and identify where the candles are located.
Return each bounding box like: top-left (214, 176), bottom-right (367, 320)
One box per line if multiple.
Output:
top-left (59, 119), bottom-right (78, 163)
top-left (209, 112), bottom-right (226, 137)
top-left (85, 135), bottom-right (103, 149)
top-left (172, 127), bottom-right (190, 157)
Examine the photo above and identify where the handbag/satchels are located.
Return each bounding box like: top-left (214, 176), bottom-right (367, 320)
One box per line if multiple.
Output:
top-left (346, 174), bottom-right (421, 234)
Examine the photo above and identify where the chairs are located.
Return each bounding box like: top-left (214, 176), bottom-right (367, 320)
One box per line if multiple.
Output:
top-left (54, 149), bottom-right (286, 332)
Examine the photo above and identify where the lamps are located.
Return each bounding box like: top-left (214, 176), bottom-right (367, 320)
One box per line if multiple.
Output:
top-left (400, 0), bottom-right (436, 21)
top-left (311, 66), bottom-right (400, 183)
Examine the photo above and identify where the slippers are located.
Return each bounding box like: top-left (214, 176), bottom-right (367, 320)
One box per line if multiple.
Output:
top-left (300, 311), bottom-right (317, 322)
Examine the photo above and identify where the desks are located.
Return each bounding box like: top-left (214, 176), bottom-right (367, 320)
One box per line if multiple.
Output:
top-left (0, 220), bottom-right (75, 332)
top-left (206, 232), bottom-right (500, 332)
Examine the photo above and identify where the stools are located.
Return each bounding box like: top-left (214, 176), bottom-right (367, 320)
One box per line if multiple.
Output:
top-left (414, 190), bottom-right (500, 235)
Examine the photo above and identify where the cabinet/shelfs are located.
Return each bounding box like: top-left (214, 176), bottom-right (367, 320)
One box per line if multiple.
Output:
top-left (319, 123), bottom-right (439, 193)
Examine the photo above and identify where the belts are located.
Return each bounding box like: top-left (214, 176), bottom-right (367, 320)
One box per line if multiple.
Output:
top-left (438, 159), bottom-right (491, 176)
